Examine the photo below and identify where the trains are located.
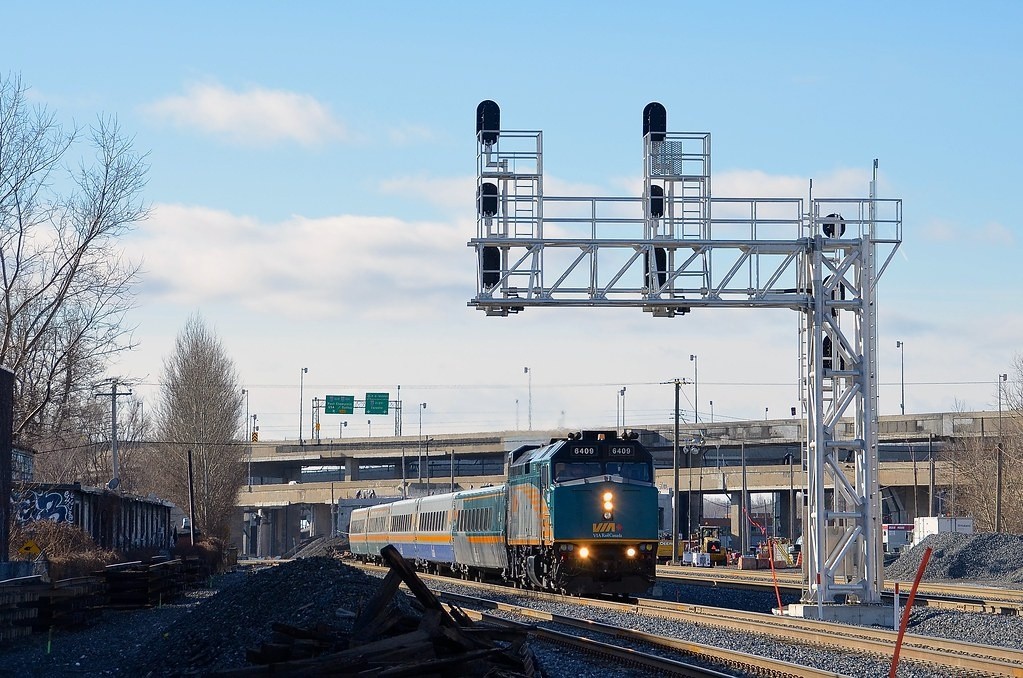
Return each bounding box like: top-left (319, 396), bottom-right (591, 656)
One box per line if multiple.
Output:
top-left (346, 428), bottom-right (667, 601)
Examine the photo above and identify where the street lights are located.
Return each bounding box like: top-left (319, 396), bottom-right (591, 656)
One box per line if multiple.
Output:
top-left (524, 366), bottom-right (532, 432)
top-left (998, 373), bottom-right (1007, 439)
top-left (397, 384), bottom-right (401, 436)
top-left (897, 340), bottom-right (905, 415)
top-left (299, 368), bottom-right (308, 441)
top-left (620, 387), bottom-right (626, 427)
top-left (689, 355), bottom-right (698, 423)
top-left (242, 388), bottom-right (251, 486)
top-left (426, 439), bottom-right (434, 496)
top-left (419, 402), bottom-right (427, 483)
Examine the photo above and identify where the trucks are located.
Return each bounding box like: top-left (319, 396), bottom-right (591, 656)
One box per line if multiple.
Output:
top-left (683, 523), bottom-right (727, 566)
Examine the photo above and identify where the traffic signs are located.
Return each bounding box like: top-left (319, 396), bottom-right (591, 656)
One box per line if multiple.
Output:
top-left (365, 393), bottom-right (389, 415)
top-left (325, 395), bottom-right (354, 415)
